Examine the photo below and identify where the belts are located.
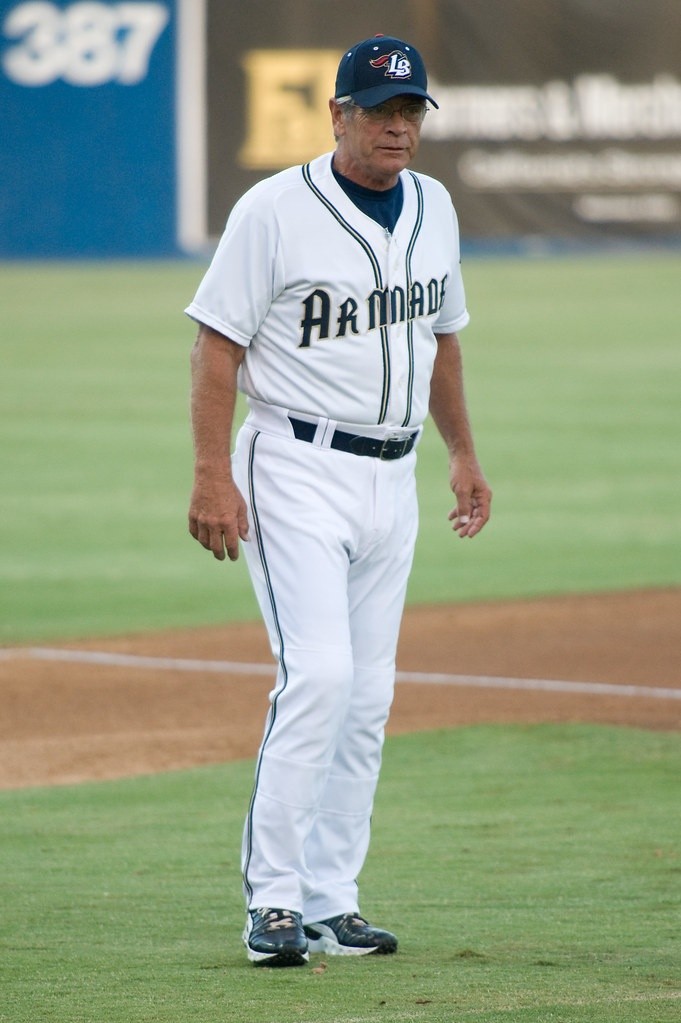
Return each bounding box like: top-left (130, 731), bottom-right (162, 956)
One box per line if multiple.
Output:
top-left (287, 416), bottom-right (421, 461)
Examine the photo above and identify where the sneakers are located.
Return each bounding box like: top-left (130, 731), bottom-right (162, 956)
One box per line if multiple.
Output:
top-left (241, 907), bottom-right (309, 966)
top-left (304, 912), bottom-right (398, 957)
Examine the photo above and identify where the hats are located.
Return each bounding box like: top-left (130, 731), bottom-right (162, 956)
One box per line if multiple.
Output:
top-left (334, 33), bottom-right (440, 111)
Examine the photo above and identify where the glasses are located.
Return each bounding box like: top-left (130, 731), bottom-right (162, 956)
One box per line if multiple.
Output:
top-left (345, 101), bottom-right (431, 123)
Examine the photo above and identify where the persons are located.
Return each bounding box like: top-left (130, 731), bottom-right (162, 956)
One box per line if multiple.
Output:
top-left (183, 33), bottom-right (492, 964)
